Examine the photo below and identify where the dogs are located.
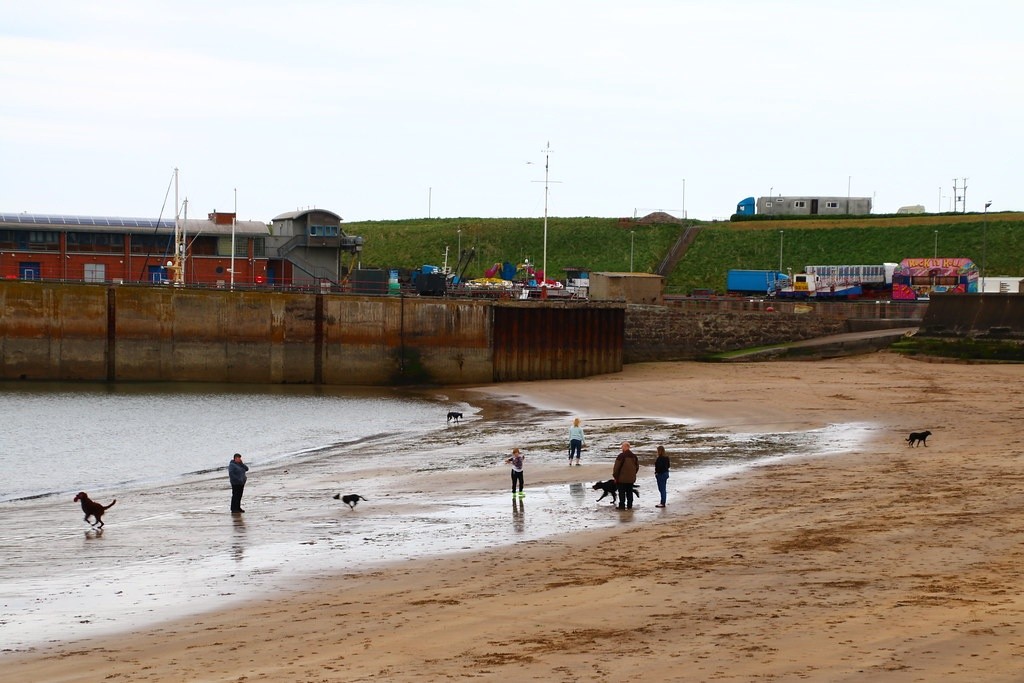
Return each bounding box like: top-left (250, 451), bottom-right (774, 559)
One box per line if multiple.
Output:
top-left (445, 411), bottom-right (464, 424)
top-left (904, 429), bottom-right (934, 448)
top-left (591, 479), bottom-right (641, 504)
top-left (332, 491), bottom-right (370, 512)
top-left (73, 490), bottom-right (117, 529)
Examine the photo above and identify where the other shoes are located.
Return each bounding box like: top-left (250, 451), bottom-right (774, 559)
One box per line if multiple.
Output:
top-left (616, 507), bottom-right (625, 510)
top-left (576, 464), bottom-right (582, 466)
top-left (232, 508), bottom-right (246, 513)
top-left (656, 504), bottom-right (665, 507)
top-left (570, 463), bottom-right (572, 465)
top-left (519, 491), bottom-right (526, 495)
top-left (513, 493), bottom-right (516, 497)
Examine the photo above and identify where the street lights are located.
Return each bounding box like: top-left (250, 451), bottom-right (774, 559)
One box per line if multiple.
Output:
top-left (769, 186), bottom-right (773, 216)
top-left (935, 230), bottom-right (938, 259)
top-left (847, 176), bottom-right (852, 214)
top-left (779, 231), bottom-right (784, 273)
top-left (939, 187), bottom-right (941, 213)
top-left (630, 231), bottom-right (634, 273)
top-left (682, 179), bottom-right (685, 220)
top-left (428, 186), bottom-right (432, 219)
top-left (457, 230), bottom-right (461, 273)
top-left (982, 203), bottom-right (992, 293)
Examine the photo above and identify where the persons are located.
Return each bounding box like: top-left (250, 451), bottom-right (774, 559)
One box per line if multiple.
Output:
top-left (767, 286), bottom-right (772, 300)
top-left (613, 442), bottom-right (639, 510)
top-left (228, 453), bottom-right (249, 512)
top-left (505, 448), bottom-right (526, 498)
top-left (568, 418), bottom-right (586, 466)
top-left (655, 446), bottom-right (670, 508)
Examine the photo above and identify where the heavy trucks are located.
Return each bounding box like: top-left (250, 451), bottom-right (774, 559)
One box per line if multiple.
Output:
top-left (775, 263), bottom-right (898, 301)
top-left (737, 196), bottom-right (873, 215)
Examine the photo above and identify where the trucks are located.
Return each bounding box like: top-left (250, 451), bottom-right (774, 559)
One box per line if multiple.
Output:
top-left (728, 269), bottom-right (792, 296)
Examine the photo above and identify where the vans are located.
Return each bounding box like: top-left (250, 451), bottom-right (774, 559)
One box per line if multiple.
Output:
top-left (897, 205), bottom-right (925, 214)
